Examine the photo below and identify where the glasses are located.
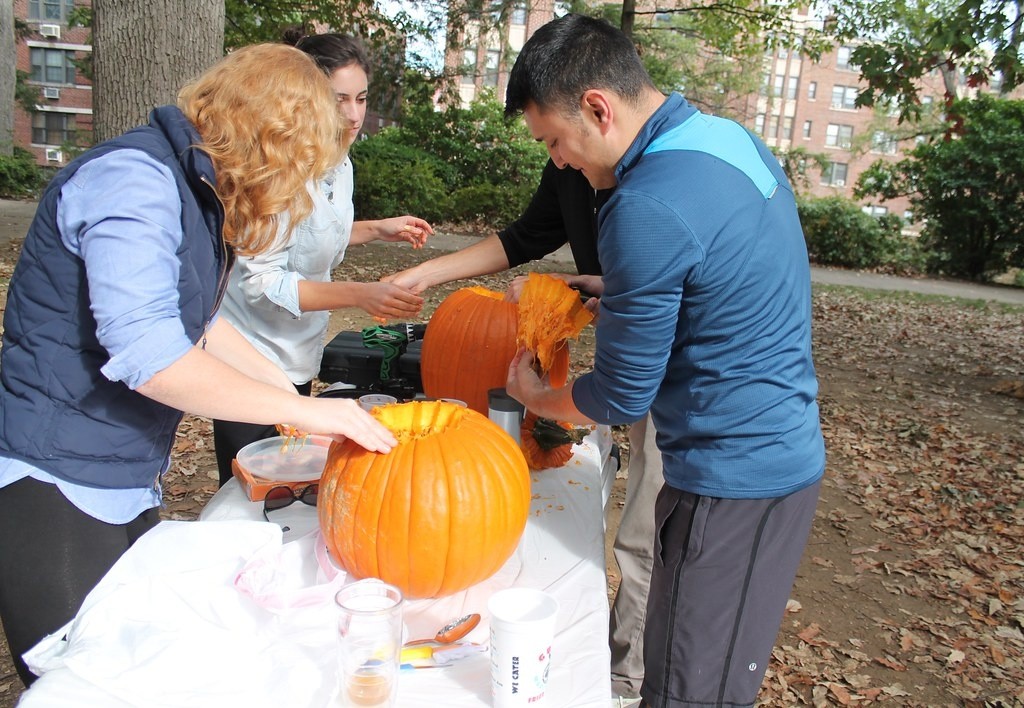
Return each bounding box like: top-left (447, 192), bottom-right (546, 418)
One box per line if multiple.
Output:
top-left (262, 483), bottom-right (319, 532)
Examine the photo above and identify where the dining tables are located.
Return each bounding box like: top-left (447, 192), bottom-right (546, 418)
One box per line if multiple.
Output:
top-left (14, 420), bottom-right (612, 708)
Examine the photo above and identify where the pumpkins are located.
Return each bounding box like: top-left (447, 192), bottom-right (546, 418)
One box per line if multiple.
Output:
top-left (315, 271), bottom-right (597, 603)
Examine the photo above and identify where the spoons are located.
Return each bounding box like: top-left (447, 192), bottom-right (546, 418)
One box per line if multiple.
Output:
top-left (401, 612), bottom-right (481, 646)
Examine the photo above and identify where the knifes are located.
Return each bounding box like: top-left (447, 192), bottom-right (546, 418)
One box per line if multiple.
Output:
top-left (398, 641), bottom-right (489, 666)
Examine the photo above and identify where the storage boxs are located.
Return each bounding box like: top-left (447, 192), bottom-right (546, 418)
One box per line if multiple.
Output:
top-left (318, 330), bottom-right (406, 392)
top-left (399, 341), bottom-right (426, 394)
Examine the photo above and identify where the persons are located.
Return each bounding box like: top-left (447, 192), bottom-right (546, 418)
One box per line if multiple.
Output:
top-left (213, 31), bottom-right (434, 487)
top-left (377, 154), bottom-right (668, 708)
top-left (504, 13), bottom-right (825, 708)
top-left (1, 42), bottom-right (399, 693)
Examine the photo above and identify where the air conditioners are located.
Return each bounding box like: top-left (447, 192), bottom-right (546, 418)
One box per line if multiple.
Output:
top-left (46, 149), bottom-right (62, 163)
top-left (39, 24), bottom-right (61, 39)
top-left (43, 87), bottom-right (60, 99)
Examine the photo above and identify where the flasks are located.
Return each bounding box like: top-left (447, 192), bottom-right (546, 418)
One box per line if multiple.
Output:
top-left (486, 388), bottom-right (524, 451)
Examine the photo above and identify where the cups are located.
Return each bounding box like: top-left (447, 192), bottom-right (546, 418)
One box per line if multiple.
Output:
top-left (332, 580), bottom-right (407, 708)
top-left (360, 394), bottom-right (398, 411)
top-left (484, 585), bottom-right (560, 708)
